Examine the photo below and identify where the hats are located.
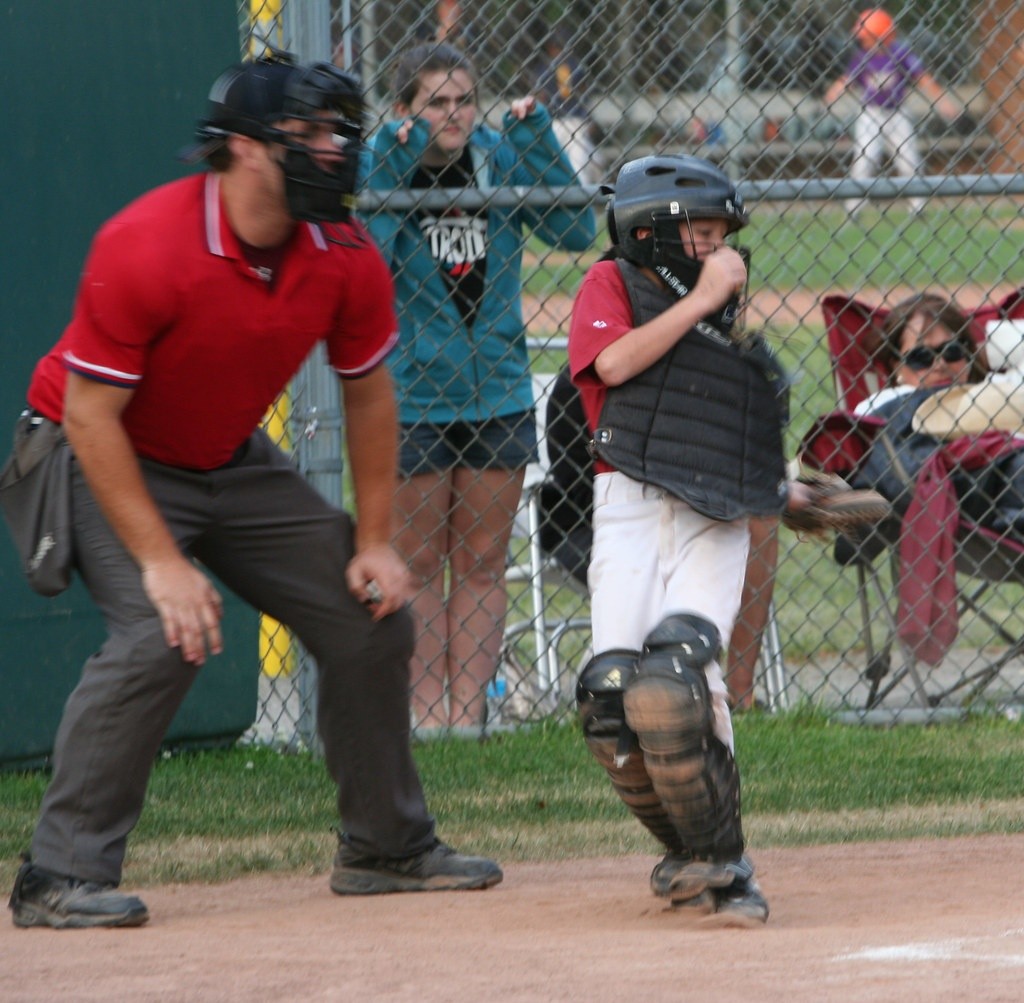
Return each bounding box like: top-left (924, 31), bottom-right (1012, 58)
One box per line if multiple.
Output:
top-left (912, 372), bottom-right (1024, 443)
top-left (192, 54), bottom-right (329, 156)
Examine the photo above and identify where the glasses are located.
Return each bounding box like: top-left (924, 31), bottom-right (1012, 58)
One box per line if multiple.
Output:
top-left (895, 338), bottom-right (971, 371)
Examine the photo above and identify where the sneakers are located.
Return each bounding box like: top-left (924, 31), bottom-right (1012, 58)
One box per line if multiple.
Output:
top-left (328, 821), bottom-right (503, 895)
top-left (10, 853), bottom-right (148, 932)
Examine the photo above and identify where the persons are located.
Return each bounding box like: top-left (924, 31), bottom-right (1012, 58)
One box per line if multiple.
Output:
top-left (354, 42), bottom-right (596, 740)
top-left (568, 153), bottom-right (788, 924)
top-left (824, 8), bottom-right (959, 222)
top-left (7, 51), bottom-right (503, 930)
top-left (532, 33), bottom-right (590, 150)
top-left (854, 293), bottom-right (982, 417)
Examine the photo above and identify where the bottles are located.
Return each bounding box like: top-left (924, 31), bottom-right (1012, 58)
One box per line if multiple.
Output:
top-left (485, 666), bottom-right (508, 723)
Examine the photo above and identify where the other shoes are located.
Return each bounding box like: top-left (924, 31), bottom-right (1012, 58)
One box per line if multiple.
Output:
top-left (671, 879), bottom-right (768, 923)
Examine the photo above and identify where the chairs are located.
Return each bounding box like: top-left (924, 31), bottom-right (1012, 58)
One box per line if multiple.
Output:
top-left (797, 288), bottom-right (1024, 722)
top-left (492, 357), bottom-right (783, 722)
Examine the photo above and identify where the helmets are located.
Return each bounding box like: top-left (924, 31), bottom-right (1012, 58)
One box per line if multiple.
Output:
top-left (604, 157), bottom-right (752, 334)
top-left (854, 11), bottom-right (896, 51)
